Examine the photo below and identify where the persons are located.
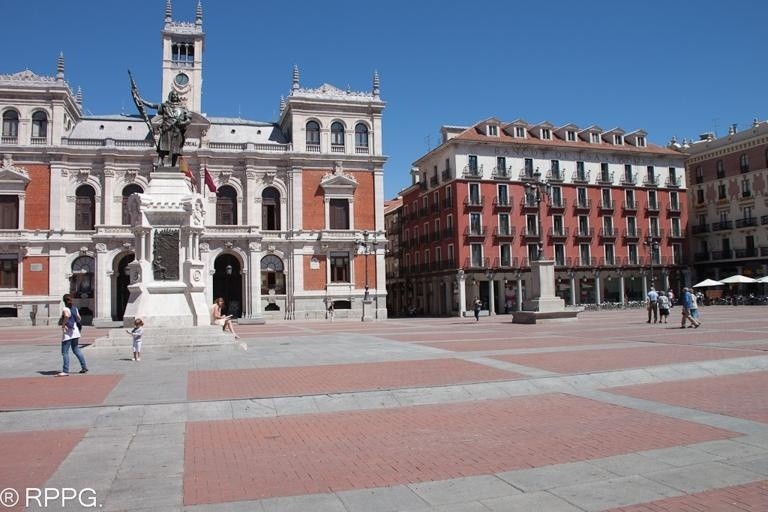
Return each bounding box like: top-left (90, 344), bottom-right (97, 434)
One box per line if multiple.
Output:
top-left (55, 292), bottom-right (89, 377)
top-left (210, 296), bottom-right (241, 340)
top-left (124, 318), bottom-right (145, 363)
top-left (327, 301), bottom-right (337, 322)
top-left (679, 287), bottom-right (700, 329)
top-left (133, 86), bottom-right (193, 168)
top-left (644, 285), bottom-right (675, 324)
top-left (473, 295), bottom-right (482, 321)
top-left (687, 288), bottom-right (702, 328)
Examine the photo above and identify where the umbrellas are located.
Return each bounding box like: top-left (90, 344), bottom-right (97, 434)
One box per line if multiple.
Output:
top-left (692, 278), bottom-right (724, 298)
top-left (756, 275), bottom-right (768, 284)
top-left (719, 275), bottom-right (759, 297)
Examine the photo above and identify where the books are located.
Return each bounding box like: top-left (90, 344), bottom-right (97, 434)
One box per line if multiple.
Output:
top-left (222, 313), bottom-right (233, 320)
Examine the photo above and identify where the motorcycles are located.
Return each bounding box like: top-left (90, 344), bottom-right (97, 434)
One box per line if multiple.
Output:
top-left (694, 292), bottom-right (767, 306)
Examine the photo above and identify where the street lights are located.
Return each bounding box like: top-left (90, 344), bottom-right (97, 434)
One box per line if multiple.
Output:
top-left (354, 229), bottom-right (379, 320)
top-left (523, 164), bottom-right (552, 261)
top-left (643, 232), bottom-right (657, 291)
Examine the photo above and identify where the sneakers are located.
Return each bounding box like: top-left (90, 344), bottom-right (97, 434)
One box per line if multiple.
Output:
top-left (680, 323), bottom-right (701, 329)
top-left (132, 358), bottom-right (141, 361)
top-left (79, 369), bottom-right (87, 373)
top-left (647, 320), bottom-right (667, 324)
top-left (235, 336), bottom-right (240, 339)
top-left (58, 372), bottom-right (68, 377)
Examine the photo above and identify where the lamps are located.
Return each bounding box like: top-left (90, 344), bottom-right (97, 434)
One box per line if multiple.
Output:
top-left (502, 272), bottom-right (508, 284)
top-left (471, 272), bottom-right (477, 286)
top-left (123, 252), bottom-right (130, 276)
top-left (557, 267), bottom-right (659, 284)
top-left (226, 264), bottom-right (233, 276)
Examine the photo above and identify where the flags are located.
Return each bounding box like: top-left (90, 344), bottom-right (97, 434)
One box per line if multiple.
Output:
top-left (203, 165), bottom-right (216, 193)
top-left (180, 155), bottom-right (197, 186)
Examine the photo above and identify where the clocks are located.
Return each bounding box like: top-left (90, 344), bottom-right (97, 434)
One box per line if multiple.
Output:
top-left (173, 71), bottom-right (191, 88)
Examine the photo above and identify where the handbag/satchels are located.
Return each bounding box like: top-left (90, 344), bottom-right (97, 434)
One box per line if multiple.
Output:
top-left (77, 321), bottom-right (81, 332)
